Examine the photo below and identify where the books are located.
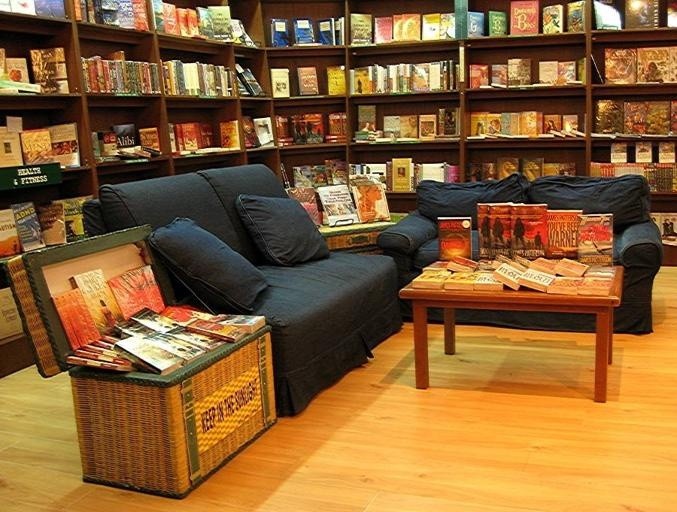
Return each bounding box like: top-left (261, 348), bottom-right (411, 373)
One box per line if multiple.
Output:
top-left (466, 1), bottom-right (586, 182)
top-left (412, 202), bottom-right (616, 296)
top-left (270, 17), bottom-right (392, 227)
top-left (590, 1), bottom-right (676, 247)
top-left (0, 195), bottom-right (265, 375)
top-left (349, 13), bottom-right (461, 193)
top-left (152, 1), bottom-right (275, 156)
top-left (0, 1), bottom-right (162, 170)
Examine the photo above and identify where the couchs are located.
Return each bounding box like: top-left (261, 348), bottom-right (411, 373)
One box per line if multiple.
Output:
top-left (80, 164), bottom-right (397, 418)
